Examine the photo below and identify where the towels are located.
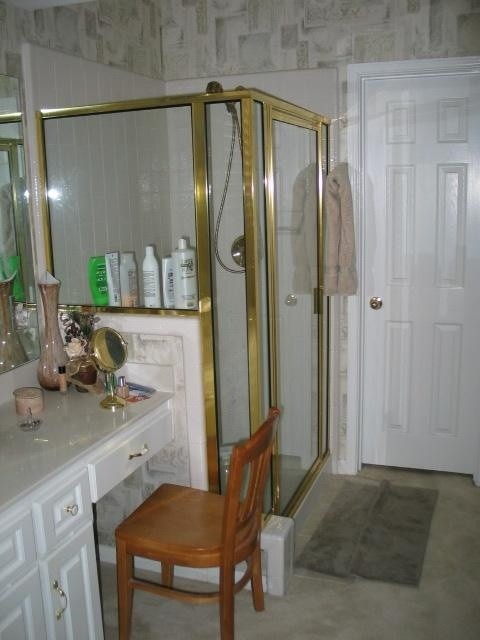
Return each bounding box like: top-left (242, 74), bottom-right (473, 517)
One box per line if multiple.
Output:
top-left (320, 161), bottom-right (359, 296)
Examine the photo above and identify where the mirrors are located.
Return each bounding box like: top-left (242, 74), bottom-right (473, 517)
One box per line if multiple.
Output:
top-left (82, 327), bottom-right (129, 411)
top-left (0, 73), bottom-right (45, 377)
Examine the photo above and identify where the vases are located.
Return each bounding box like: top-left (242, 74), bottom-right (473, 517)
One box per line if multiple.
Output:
top-left (0, 269), bottom-right (27, 374)
top-left (37, 282), bottom-right (72, 391)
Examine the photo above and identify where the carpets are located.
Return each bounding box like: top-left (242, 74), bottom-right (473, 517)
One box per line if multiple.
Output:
top-left (294, 480), bottom-right (442, 587)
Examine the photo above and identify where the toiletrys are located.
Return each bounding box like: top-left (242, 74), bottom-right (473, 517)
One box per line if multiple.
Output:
top-left (116, 376), bottom-right (129, 398)
top-left (56, 364), bottom-right (70, 393)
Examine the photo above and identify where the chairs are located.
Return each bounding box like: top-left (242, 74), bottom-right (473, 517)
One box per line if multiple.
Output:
top-left (111, 405), bottom-right (282, 640)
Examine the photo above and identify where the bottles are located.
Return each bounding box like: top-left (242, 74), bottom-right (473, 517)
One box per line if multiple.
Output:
top-left (171, 238), bottom-right (199, 311)
top-left (105, 373), bottom-right (117, 396)
top-left (141, 246), bottom-right (162, 309)
top-left (119, 252), bottom-right (140, 308)
top-left (58, 366), bottom-right (67, 393)
top-left (162, 258), bottom-right (174, 309)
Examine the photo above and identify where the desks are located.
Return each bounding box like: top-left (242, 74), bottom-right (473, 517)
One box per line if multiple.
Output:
top-left (0, 380), bottom-right (174, 639)
